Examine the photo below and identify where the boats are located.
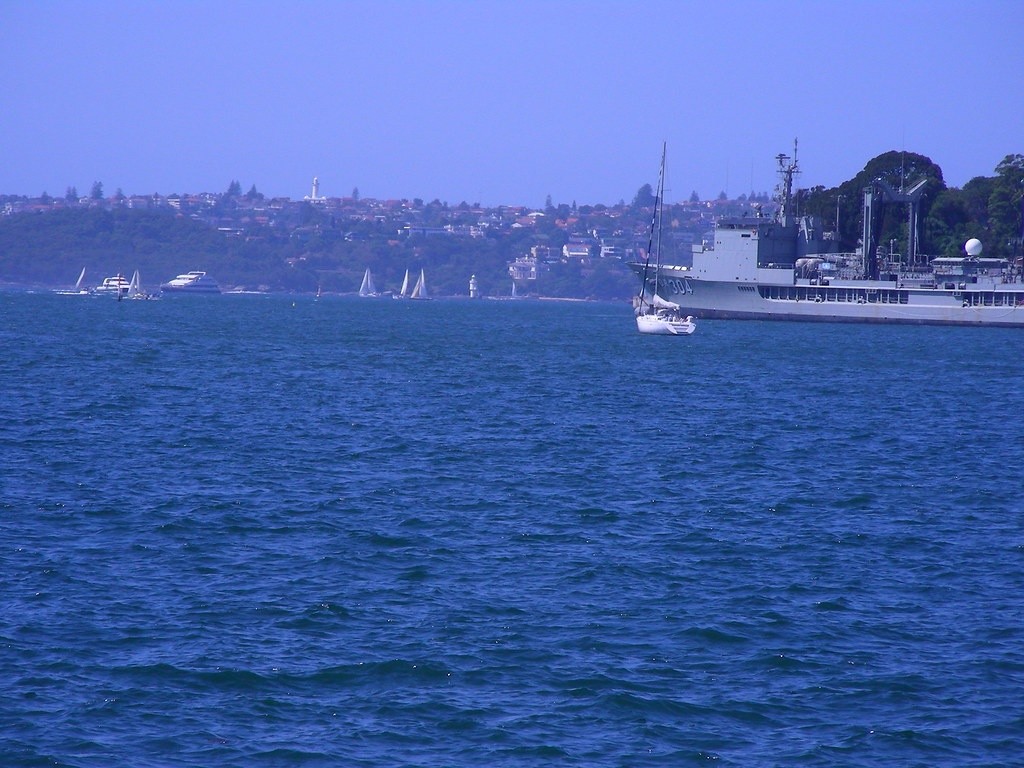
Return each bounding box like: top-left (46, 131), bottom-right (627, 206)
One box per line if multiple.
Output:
top-left (95, 276), bottom-right (137, 293)
top-left (159, 269), bottom-right (220, 294)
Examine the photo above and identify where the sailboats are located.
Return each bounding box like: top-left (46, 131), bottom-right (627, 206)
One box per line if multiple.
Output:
top-left (121, 269), bottom-right (164, 300)
top-left (636, 139), bottom-right (698, 335)
top-left (508, 281), bottom-right (524, 300)
top-left (230, 264), bottom-right (430, 301)
top-left (54, 267), bottom-right (94, 295)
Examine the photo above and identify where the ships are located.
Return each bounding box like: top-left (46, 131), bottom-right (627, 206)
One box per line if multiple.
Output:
top-left (626, 136), bottom-right (1023, 328)
